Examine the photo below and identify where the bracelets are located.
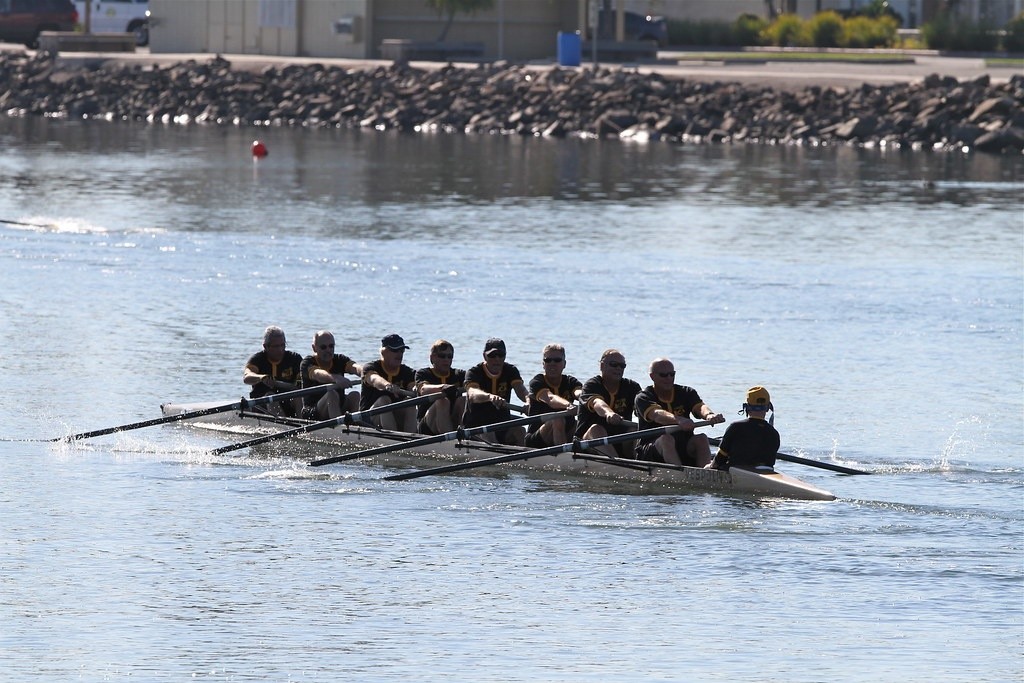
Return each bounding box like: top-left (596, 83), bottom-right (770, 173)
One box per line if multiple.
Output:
top-left (704, 412), bottom-right (714, 419)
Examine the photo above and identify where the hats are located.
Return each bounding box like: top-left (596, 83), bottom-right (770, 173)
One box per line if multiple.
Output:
top-left (747, 386), bottom-right (770, 406)
top-left (382, 334), bottom-right (410, 350)
top-left (484, 338), bottom-right (505, 355)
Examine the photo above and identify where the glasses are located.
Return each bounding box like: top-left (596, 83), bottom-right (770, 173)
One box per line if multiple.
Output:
top-left (434, 353), bottom-right (453, 359)
top-left (386, 347), bottom-right (405, 353)
top-left (487, 353), bottom-right (506, 358)
top-left (316, 344), bottom-right (334, 350)
top-left (605, 361), bottom-right (626, 369)
top-left (654, 371), bottom-right (675, 377)
top-left (543, 358), bottom-right (564, 363)
top-left (267, 343), bottom-right (286, 349)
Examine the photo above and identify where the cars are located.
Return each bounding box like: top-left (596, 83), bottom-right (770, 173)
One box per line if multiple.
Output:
top-left (0, 0), bottom-right (78, 50)
top-left (68, 0), bottom-right (149, 48)
top-left (597, 7), bottom-right (669, 51)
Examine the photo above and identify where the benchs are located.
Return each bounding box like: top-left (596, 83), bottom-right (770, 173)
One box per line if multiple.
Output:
top-left (37, 31), bottom-right (136, 53)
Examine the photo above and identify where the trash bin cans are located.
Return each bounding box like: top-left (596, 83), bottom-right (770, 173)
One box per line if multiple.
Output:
top-left (557, 31), bottom-right (582, 67)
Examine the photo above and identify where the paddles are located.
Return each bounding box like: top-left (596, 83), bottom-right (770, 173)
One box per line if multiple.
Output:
top-left (208, 392), bottom-right (443, 456)
top-left (384, 421), bottom-right (711, 479)
top-left (677, 423), bottom-right (876, 479)
top-left (57, 377), bottom-right (362, 441)
top-left (309, 410), bottom-right (567, 466)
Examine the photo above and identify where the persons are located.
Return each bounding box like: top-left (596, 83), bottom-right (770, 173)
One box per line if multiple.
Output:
top-left (703, 385), bottom-right (780, 471)
top-left (300, 330), bottom-right (362, 419)
top-left (524, 344), bottom-right (583, 446)
top-left (243, 326), bottom-right (304, 419)
top-left (361, 333), bottom-right (417, 432)
top-left (462, 338), bottom-right (529, 446)
top-left (415, 339), bottom-right (466, 436)
top-left (634, 358), bottom-right (723, 468)
top-left (572, 348), bottom-right (642, 458)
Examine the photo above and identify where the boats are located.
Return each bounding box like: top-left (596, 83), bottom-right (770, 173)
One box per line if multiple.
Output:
top-left (160, 396), bottom-right (836, 502)
